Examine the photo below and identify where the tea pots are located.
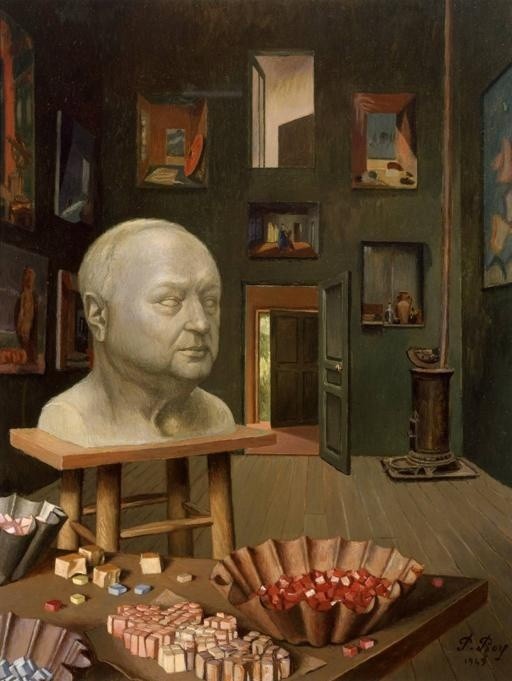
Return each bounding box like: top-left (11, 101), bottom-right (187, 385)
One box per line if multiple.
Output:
top-left (245, 48), bottom-right (318, 173)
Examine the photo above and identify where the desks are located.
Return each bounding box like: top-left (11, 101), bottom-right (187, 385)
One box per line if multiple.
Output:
top-left (0, 426), bottom-right (491, 681)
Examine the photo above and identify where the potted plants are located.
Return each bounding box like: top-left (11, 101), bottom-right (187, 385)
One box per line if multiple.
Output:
top-left (350, 90), bottom-right (418, 192)
top-left (247, 199), bottom-right (319, 260)
top-left (358, 239), bottom-right (428, 331)
top-left (480, 60), bottom-right (510, 291)
top-left (136, 89), bottom-right (212, 194)
top-left (53, 267), bottom-right (92, 373)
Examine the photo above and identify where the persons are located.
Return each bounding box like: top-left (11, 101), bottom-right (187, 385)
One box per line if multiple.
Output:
top-left (277, 224), bottom-right (289, 250)
top-left (14, 267), bottom-right (38, 360)
top-left (385, 301), bottom-right (399, 325)
top-left (37, 217), bottom-right (237, 448)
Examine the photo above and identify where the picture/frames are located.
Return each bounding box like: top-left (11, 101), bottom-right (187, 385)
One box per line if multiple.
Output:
top-left (480, 60), bottom-right (510, 291)
top-left (53, 267), bottom-right (92, 373)
top-left (247, 199), bottom-right (319, 260)
top-left (350, 90), bottom-right (418, 192)
top-left (136, 89), bottom-right (212, 194)
top-left (358, 239), bottom-right (428, 331)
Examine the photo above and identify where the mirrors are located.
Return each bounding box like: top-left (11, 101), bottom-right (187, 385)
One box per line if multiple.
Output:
top-left (245, 48), bottom-right (318, 173)
